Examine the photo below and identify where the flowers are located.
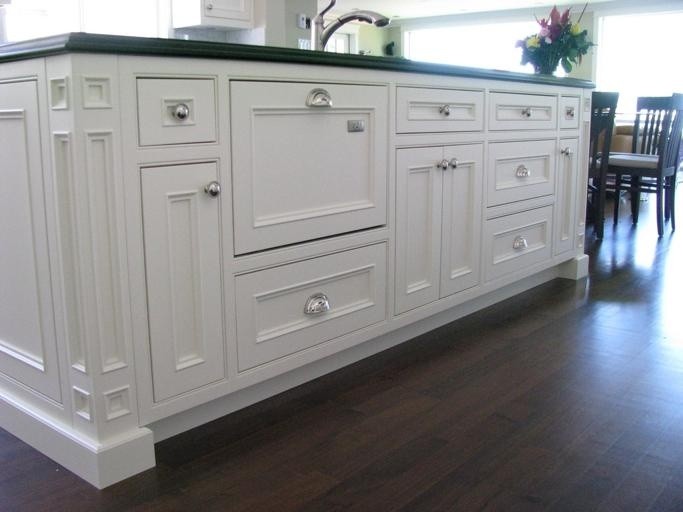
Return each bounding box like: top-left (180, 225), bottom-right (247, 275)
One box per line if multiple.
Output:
top-left (515, 4), bottom-right (596, 77)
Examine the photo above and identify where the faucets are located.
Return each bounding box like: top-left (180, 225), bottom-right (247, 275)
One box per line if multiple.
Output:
top-left (308, 1), bottom-right (389, 54)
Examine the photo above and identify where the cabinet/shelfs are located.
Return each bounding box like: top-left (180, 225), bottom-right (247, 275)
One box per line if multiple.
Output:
top-left (172, 0), bottom-right (254, 32)
top-left (1, 53), bottom-right (592, 489)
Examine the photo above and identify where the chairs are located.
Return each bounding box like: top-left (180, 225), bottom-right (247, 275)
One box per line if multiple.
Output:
top-left (586, 91), bottom-right (683, 239)
